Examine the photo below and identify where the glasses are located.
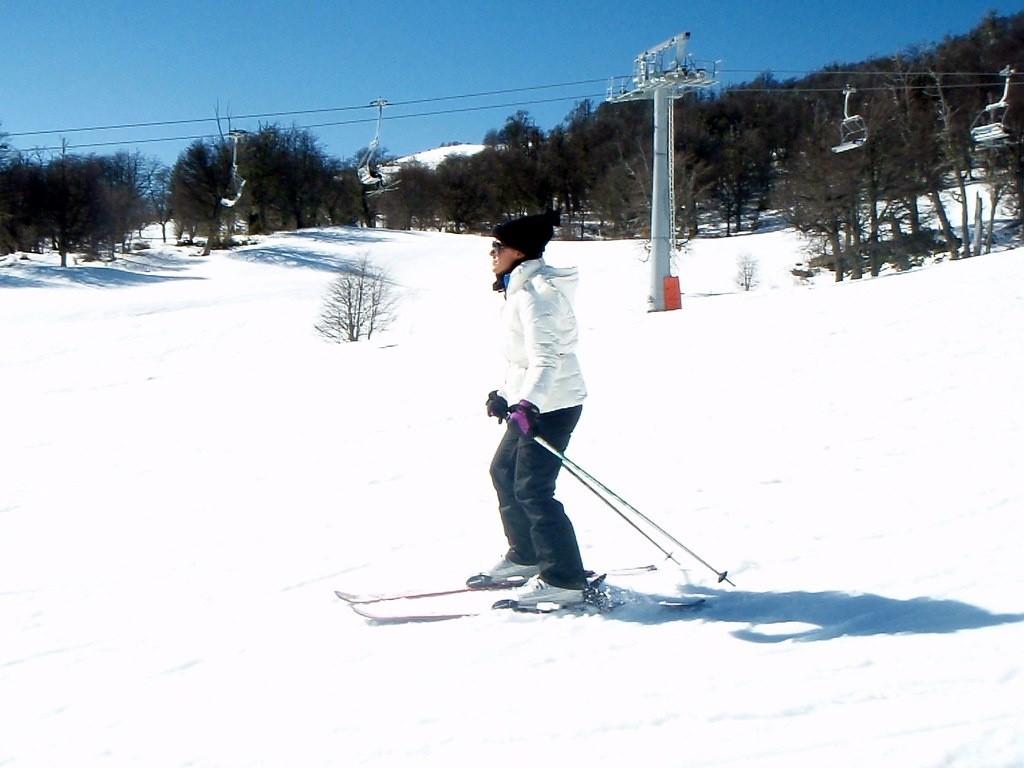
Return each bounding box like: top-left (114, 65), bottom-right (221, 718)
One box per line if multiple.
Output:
top-left (492, 241), bottom-right (508, 254)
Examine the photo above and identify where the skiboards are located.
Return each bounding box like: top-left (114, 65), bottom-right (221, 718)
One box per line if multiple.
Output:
top-left (334, 564), bottom-right (706, 624)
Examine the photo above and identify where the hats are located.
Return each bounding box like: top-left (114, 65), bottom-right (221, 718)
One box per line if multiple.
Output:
top-left (493, 208), bottom-right (561, 260)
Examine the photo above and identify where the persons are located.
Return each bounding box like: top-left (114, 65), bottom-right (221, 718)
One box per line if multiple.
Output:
top-left (359, 162), bottom-right (383, 190)
top-left (480, 217), bottom-right (589, 605)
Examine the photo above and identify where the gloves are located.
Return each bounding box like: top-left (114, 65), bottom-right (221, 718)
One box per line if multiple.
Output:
top-left (507, 401), bottom-right (541, 444)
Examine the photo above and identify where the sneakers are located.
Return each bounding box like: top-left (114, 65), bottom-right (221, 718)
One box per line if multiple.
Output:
top-left (482, 557), bottom-right (539, 583)
top-left (514, 577), bottom-right (584, 605)
